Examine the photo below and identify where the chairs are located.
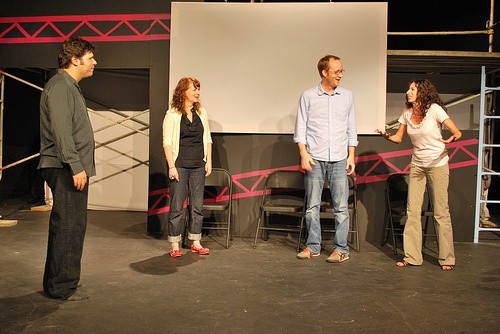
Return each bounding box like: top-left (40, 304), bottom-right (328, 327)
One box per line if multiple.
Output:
top-left (321, 175), bottom-right (359, 251)
top-left (182, 168), bottom-right (231, 248)
top-left (382, 173), bottom-right (438, 256)
top-left (253, 170), bottom-right (306, 251)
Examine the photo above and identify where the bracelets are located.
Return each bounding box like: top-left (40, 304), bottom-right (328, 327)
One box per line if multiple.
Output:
top-left (169, 166), bottom-right (175, 169)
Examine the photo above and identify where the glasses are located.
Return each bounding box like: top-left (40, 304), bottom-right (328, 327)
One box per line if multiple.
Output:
top-left (328, 68), bottom-right (344, 75)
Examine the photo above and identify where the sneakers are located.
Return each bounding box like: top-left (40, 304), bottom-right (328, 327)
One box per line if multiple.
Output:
top-left (327, 249), bottom-right (349, 263)
top-left (297, 248), bottom-right (321, 259)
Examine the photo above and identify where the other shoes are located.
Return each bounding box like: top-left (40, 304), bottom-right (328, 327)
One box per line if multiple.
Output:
top-left (191, 245), bottom-right (209, 255)
top-left (169, 248), bottom-right (182, 257)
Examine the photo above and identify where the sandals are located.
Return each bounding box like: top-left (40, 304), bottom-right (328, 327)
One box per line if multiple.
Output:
top-left (396, 261), bottom-right (411, 267)
top-left (441, 265), bottom-right (455, 270)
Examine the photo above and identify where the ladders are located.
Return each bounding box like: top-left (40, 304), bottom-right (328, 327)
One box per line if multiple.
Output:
top-left (474, 63), bottom-right (500, 246)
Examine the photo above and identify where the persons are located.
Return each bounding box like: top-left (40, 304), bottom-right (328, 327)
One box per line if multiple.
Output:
top-left (38, 39), bottom-right (96, 301)
top-left (163, 77), bottom-right (212, 257)
top-left (293, 54), bottom-right (359, 262)
top-left (374, 78), bottom-right (461, 271)
